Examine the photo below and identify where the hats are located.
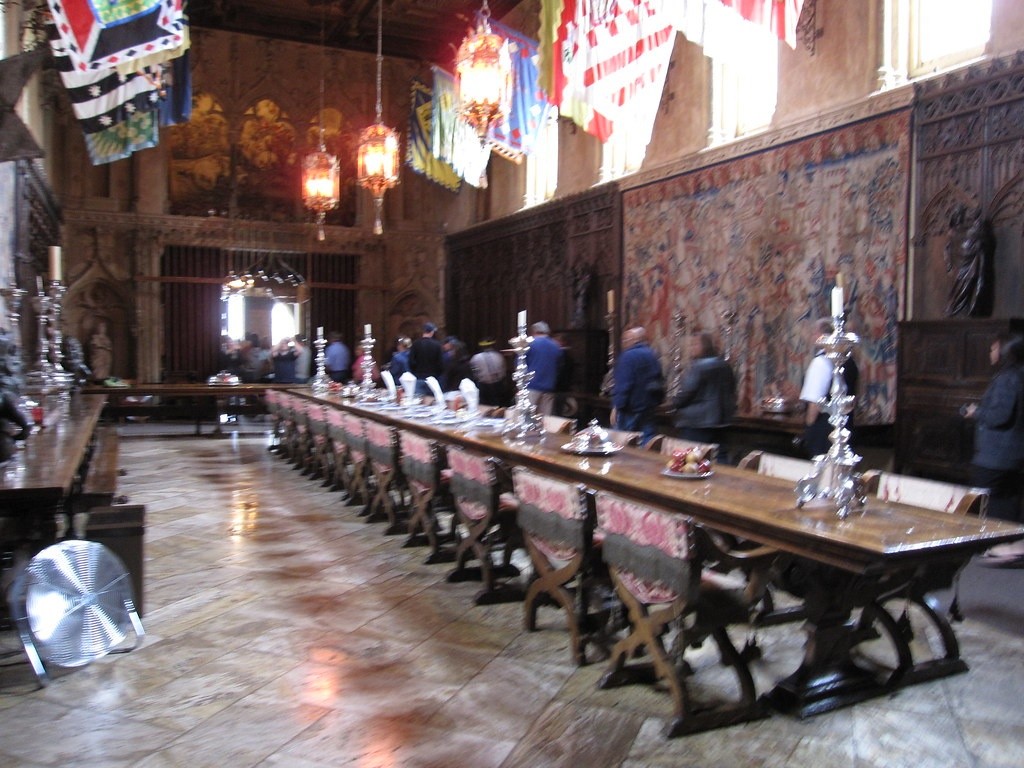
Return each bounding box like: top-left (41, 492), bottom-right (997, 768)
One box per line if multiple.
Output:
top-left (530, 320), bottom-right (551, 334)
top-left (325, 330), bottom-right (342, 338)
top-left (247, 333), bottom-right (258, 341)
top-left (290, 334), bottom-right (307, 342)
top-left (421, 322), bottom-right (435, 331)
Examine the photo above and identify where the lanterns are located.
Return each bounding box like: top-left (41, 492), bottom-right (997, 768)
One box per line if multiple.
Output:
top-left (301, 148), bottom-right (341, 242)
top-left (453, 26), bottom-right (504, 153)
top-left (354, 124), bottom-right (400, 237)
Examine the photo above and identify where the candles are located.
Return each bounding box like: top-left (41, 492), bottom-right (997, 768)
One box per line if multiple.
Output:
top-left (608, 292), bottom-right (614, 313)
top-left (364, 324), bottom-right (371, 334)
top-left (832, 287), bottom-right (843, 318)
top-left (518, 309), bottom-right (526, 327)
top-left (49, 246), bottom-right (61, 280)
top-left (317, 327), bottom-right (323, 339)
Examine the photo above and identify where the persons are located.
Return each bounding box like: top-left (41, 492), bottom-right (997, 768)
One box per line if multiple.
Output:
top-left (387, 323), bottom-right (508, 405)
top-left (802, 318), bottom-right (858, 461)
top-left (963, 334), bottom-right (1024, 557)
top-left (943, 205), bottom-right (984, 318)
top-left (220, 332), bottom-right (382, 423)
top-left (516, 319), bottom-right (583, 417)
top-left (655, 334), bottom-right (736, 464)
top-left (609, 328), bottom-right (665, 448)
top-left (89, 324), bottom-right (113, 379)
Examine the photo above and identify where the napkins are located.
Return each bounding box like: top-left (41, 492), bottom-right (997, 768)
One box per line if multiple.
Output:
top-left (383, 405), bottom-right (406, 411)
top-left (399, 371), bottom-right (417, 395)
top-left (401, 413), bottom-right (433, 418)
top-left (458, 378), bottom-right (480, 411)
top-left (425, 376), bottom-right (444, 402)
top-left (357, 400), bottom-right (385, 407)
top-left (428, 418), bottom-right (464, 427)
top-left (475, 417), bottom-right (507, 428)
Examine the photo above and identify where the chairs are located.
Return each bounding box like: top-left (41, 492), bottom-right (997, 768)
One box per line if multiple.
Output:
top-left (641, 432), bottom-right (718, 468)
top-left (265, 388), bottom-right (772, 741)
top-left (852, 463), bottom-right (992, 640)
top-left (725, 441), bottom-right (827, 626)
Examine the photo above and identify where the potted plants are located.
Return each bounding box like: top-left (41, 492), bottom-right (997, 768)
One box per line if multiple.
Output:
top-left (381, 370), bottom-right (396, 395)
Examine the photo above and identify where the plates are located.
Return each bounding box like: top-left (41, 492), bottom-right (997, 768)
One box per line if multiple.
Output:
top-left (561, 442), bottom-right (624, 453)
top-left (314, 384), bottom-right (507, 426)
top-left (660, 468), bottom-right (715, 478)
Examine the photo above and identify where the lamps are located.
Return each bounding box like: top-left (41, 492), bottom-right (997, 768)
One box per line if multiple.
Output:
top-left (356, 0), bottom-right (400, 234)
top-left (301, 0), bottom-right (342, 241)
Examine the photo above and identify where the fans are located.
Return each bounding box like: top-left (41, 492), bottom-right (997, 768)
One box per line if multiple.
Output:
top-left (8, 540), bottom-right (145, 688)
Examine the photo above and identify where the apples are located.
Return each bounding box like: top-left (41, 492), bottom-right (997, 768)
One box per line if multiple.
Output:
top-left (666, 447), bottom-right (711, 474)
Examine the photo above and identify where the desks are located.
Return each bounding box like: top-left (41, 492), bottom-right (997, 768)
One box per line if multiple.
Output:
top-left (0, 392), bottom-right (144, 624)
top-left (287, 386), bottom-right (1024, 741)
top-left (69, 382), bottom-right (307, 434)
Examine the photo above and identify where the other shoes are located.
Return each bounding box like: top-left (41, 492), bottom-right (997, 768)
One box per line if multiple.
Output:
top-left (975, 545), bottom-right (1021, 566)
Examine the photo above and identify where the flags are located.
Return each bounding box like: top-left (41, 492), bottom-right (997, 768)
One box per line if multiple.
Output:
top-left (46, 0), bottom-right (191, 167)
top-left (404, 0), bottom-right (802, 195)
